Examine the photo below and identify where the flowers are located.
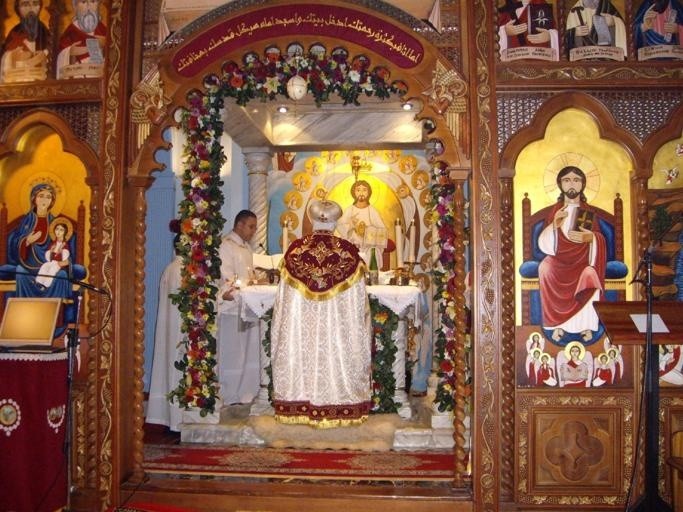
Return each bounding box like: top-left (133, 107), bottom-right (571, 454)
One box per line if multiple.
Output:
top-left (168, 52), bottom-right (473, 417)
top-left (260, 295), bottom-right (402, 414)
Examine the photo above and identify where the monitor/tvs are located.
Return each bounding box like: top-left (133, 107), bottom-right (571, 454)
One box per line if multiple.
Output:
top-left (0, 297), bottom-right (62, 346)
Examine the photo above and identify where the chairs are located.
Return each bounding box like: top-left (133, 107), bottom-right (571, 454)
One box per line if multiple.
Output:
top-left (0, 200), bottom-right (85, 330)
top-left (522, 193), bottom-right (628, 325)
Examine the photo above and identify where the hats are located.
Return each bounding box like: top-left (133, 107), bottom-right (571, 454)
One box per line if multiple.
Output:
top-left (48, 218), bottom-right (73, 242)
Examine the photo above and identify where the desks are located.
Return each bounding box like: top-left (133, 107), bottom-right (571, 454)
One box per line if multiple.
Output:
top-left (239, 284), bottom-right (422, 416)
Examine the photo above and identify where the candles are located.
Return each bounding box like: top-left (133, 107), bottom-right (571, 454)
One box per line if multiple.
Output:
top-left (395, 218), bottom-right (404, 268)
top-left (282, 223), bottom-right (288, 255)
top-left (409, 217), bottom-right (416, 262)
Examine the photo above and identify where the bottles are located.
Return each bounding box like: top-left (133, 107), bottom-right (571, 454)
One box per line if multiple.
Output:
top-left (368, 247), bottom-right (380, 287)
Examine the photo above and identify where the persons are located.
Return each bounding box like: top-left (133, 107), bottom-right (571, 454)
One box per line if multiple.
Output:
top-left (335, 180), bottom-right (389, 271)
top-left (526, 333), bottom-right (683, 389)
top-left (0, 1), bottom-right (49, 82)
top-left (536, 167), bottom-right (607, 342)
top-left (633, 2), bottom-right (683, 63)
top-left (6, 184), bottom-right (72, 296)
top-left (218, 210), bottom-right (269, 406)
top-left (146, 219), bottom-right (191, 432)
top-left (270, 200), bottom-right (372, 427)
top-left (498, 1), bottom-right (558, 61)
top-left (35, 217), bottom-right (72, 293)
top-left (57, 1), bottom-right (107, 82)
top-left (567, 1), bottom-right (627, 61)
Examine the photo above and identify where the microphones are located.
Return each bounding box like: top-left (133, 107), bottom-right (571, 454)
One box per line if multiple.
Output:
top-left (259, 243), bottom-right (273, 255)
top-left (629, 245), bottom-right (655, 281)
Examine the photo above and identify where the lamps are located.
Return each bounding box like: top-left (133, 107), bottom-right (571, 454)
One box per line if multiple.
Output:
top-left (351, 149), bottom-right (373, 181)
